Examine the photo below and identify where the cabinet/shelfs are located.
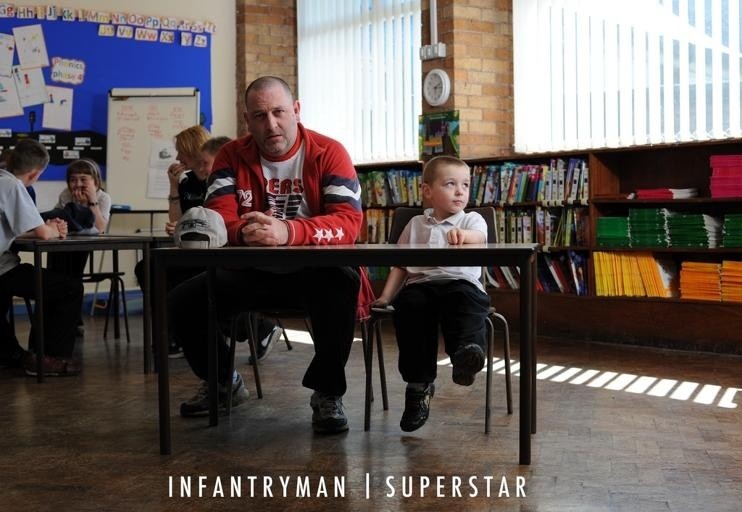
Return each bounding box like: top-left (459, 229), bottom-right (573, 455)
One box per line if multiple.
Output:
top-left (354, 134), bottom-right (741, 359)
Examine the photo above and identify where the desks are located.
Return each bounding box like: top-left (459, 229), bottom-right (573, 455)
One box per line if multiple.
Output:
top-left (150, 243), bottom-right (540, 465)
top-left (14, 235), bottom-right (175, 383)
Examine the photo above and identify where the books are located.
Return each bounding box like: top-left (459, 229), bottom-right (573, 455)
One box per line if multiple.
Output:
top-left (356, 151), bottom-right (741, 304)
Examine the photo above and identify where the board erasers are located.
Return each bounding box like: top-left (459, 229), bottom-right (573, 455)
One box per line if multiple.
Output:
top-left (111, 205), bottom-right (130, 210)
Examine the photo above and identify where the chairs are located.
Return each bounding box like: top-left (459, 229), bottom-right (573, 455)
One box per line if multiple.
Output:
top-left (364, 207), bottom-right (512, 434)
top-left (226, 303), bottom-right (389, 415)
top-left (82, 205), bottom-right (130, 343)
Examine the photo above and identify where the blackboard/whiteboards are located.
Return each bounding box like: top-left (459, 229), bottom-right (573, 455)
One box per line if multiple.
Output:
top-left (103, 88), bottom-right (201, 213)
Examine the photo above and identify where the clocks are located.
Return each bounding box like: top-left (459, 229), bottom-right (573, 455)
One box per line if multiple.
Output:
top-left (422, 68), bottom-right (451, 107)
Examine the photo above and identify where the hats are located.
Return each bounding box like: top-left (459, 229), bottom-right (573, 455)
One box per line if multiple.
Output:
top-left (173, 205), bottom-right (229, 249)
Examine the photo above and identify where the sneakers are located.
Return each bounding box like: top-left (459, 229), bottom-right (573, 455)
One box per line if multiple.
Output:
top-left (450, 343), bottom-right (486, 387)
top-left (179, 368), bottom-right (251, 418)
top-left (167, 343), bottom-right (186, 359)
top-left (400, 382), bottom-right (436, 432)
top-left (248, 326), bottom-right (283, 364)
top-left (24, 351), bottom-right (82, 377)
top-left (309, 392), bottom-right (350, 434)
top-left (0, 345), bottom-right (37, 368)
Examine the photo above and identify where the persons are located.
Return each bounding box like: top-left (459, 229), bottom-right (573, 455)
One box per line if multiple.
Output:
top-left (0, 139), bottom-right (112, 382)
top-left (371, 156), bottom-right (492, 432)
top-left (135, 77), bottom-right (377, 435)
